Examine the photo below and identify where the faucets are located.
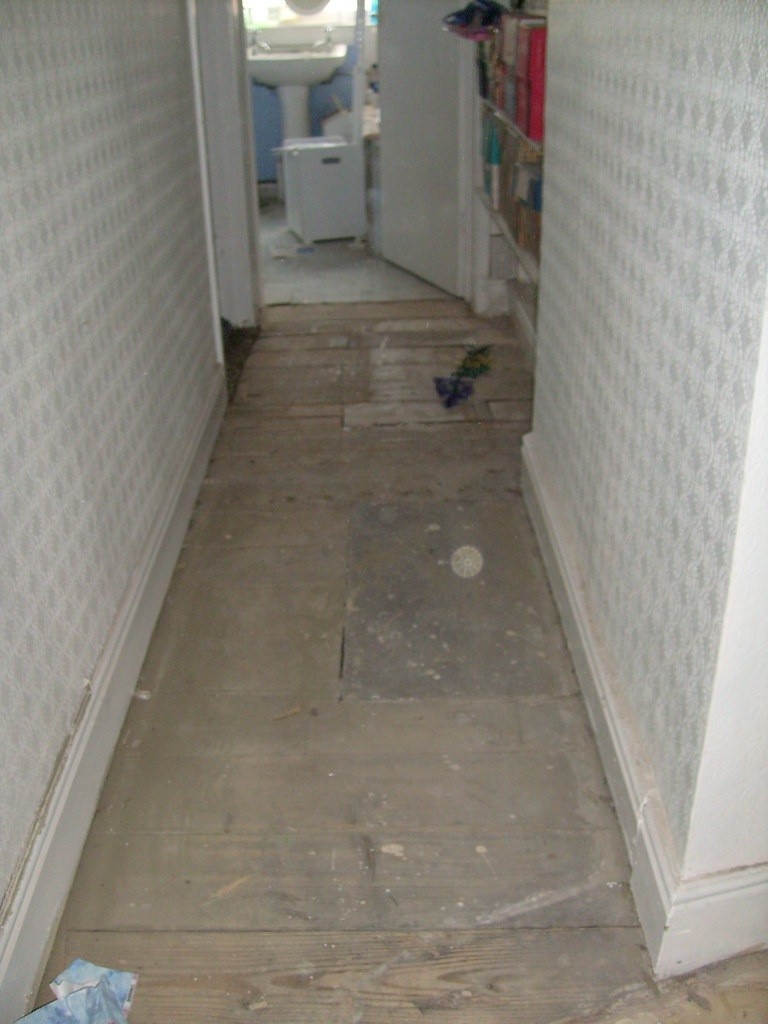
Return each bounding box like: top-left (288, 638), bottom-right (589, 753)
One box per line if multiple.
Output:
top-left (247, 27), bottom-right (271, 52)
top-left (312, 24), bottom-right (337, 51)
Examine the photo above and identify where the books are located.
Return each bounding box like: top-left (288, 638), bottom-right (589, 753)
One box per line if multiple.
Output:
top-left (480, 101), bottom-right (543, 262)
top-left (477, 12), bottom-right (547, 139)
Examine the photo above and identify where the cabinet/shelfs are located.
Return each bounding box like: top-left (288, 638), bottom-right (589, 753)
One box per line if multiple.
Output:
top-left (477, 0), bottom-right (546, 353)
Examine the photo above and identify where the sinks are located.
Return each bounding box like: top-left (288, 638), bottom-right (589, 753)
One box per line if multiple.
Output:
top-left (246, 42), bottom-right (348, 88)
